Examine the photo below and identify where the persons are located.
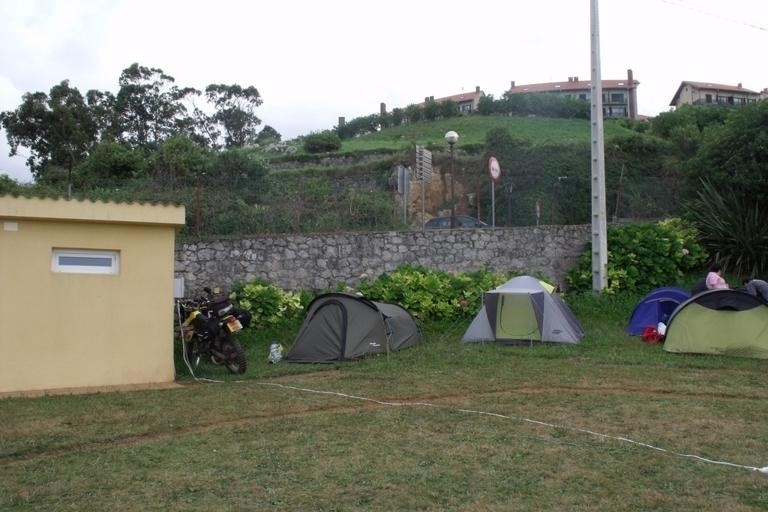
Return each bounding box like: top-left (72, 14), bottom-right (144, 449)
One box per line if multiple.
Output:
top-left (742, 278), bottom-right (768, 303)
top-left (705, 263), bottom-right (730, 290)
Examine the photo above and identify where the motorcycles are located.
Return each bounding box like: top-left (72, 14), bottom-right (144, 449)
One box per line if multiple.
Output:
top-left (174, 287), bottom-right (252, 375)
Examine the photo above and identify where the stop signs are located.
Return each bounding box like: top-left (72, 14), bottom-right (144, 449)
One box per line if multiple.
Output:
top-left (489, 155), bottom-right (501, 179)
top-left (535, 200), bottom-right (541, 219)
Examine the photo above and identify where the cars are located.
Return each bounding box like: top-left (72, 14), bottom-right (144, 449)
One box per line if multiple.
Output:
top-left (425, 215), bottom-right (489, 229)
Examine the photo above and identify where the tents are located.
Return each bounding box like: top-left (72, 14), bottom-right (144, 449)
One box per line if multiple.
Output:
top-left (662, 289), bottom-right (768, 359)
top-left (625, 286), bottom-right (692, 336)
top-left (461, 276), bottom-right (587, 348)
top-left (283, 292), bottom-right (423, 363)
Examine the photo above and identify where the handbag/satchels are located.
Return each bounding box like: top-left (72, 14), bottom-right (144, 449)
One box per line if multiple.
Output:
top-left (642, 327), bottom-right (663, 343)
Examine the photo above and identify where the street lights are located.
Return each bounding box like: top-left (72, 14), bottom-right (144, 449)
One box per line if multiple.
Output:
top-left (445, 130), bottom-right (459, 229)
